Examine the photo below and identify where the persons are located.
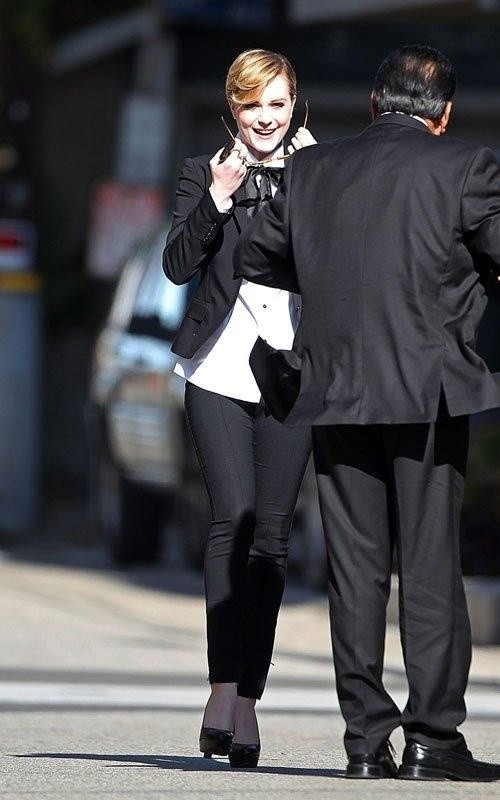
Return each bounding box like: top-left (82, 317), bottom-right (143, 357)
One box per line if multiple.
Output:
top-left (162, 45), bottom-right (319, 770)
top-left (229, 40), bottom-right (500, 785)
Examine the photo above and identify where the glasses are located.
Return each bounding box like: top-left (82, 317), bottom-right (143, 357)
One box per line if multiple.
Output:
top-left (220, 100), bottom-right (310, 165)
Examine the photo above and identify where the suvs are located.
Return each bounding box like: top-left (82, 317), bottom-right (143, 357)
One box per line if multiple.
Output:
top-left (78, 208), bottom-right (327, 588)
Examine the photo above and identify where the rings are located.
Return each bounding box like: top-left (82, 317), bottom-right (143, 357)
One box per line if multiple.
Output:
top-left (238, 155), bottom-right (247, 163)
top-left (232, 149), bottom-right (241, 154)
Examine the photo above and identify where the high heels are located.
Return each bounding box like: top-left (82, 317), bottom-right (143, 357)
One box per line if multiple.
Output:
top-left (229, 711), bottom-right (261, 767)
top-left (198, 692), bottom-right (236, 760)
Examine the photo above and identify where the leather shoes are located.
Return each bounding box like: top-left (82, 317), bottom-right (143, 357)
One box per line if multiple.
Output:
top-left (346, 742), bottom-right (397, 780)
top-left (398, 736), bottom-right (500, 781)
